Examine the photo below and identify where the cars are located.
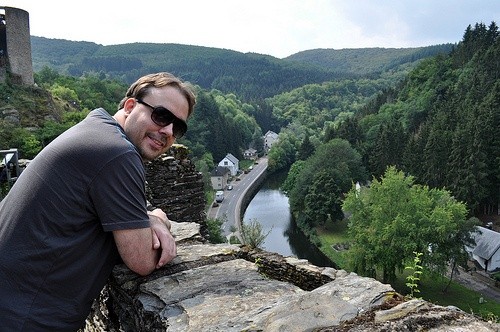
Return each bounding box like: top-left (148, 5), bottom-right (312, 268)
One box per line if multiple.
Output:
top-left (254, 161), bottom-right (259, 165)
top-left (244, 166), bottom-right (253, 174)
top-left (227, 185), bottom-right (233, 190)
top-left (236, 178), bottom-right (241, 181)
top-left (212, 202), bottom-right (218, 208)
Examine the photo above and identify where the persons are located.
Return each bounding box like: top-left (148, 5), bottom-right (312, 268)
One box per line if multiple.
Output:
top-left (0, 72), bottom-right (196, 332)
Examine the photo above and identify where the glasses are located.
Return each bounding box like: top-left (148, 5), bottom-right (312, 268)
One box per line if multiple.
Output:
top-left (136, 98), bottom-right (188, 139)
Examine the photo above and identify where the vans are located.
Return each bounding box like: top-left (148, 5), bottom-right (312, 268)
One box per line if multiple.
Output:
top-left (216, 191), bottom-right (224, 203)
